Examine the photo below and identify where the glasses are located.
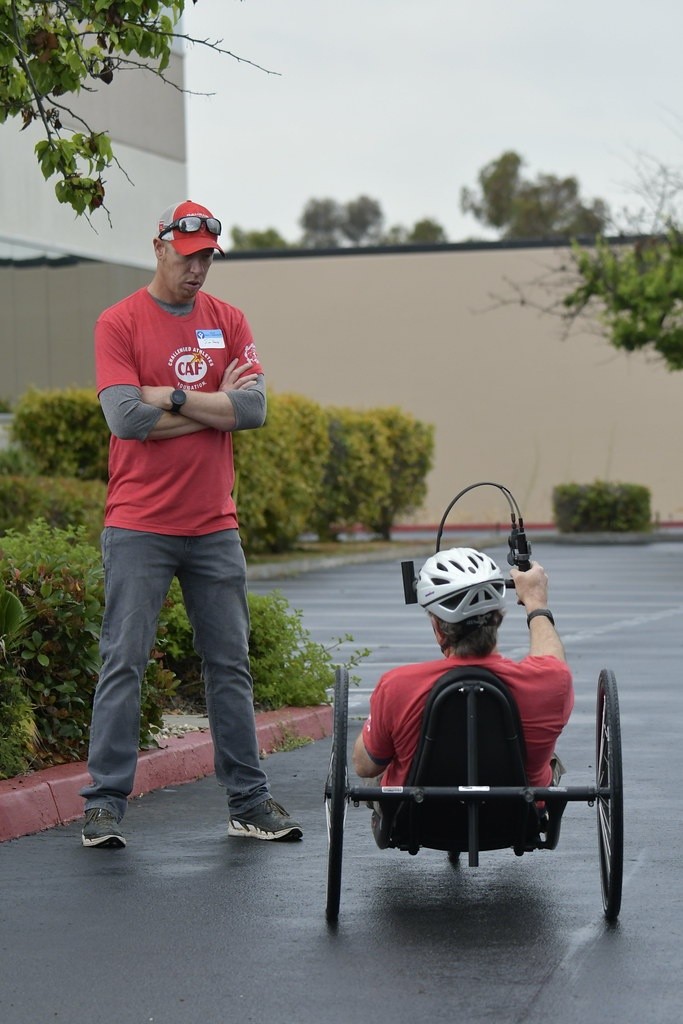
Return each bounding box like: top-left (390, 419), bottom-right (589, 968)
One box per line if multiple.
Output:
top-left (158, 216), bottom-right (221, 239)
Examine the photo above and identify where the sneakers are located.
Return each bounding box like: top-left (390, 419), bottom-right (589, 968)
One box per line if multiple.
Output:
top-left (81, 808), bottom-right (126, 848)
top-left (228, 798), bottom-right (303, 841)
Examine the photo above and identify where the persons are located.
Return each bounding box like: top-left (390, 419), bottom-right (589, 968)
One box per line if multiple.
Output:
top-left (80, 199), bottom-right (304, 848)
top-left (354, 546), bottom-right (573, 834)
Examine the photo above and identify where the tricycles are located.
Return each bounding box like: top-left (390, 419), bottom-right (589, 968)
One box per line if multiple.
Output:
top-left (322, 481), bottom-right (626, 921)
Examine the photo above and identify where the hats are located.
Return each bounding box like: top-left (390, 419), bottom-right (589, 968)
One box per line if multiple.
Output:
top-left (155, 199), bottom-right (226, 258)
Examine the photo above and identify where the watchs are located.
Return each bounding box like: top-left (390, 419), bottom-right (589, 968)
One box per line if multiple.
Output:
top-left (169, 389), bottom-right (186, 414)
top-left (527, 608), bottom-right (555, 629)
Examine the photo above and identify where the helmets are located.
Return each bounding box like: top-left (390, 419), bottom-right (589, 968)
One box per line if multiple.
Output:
top-left (416, 547), bottom-right (508, 624)
